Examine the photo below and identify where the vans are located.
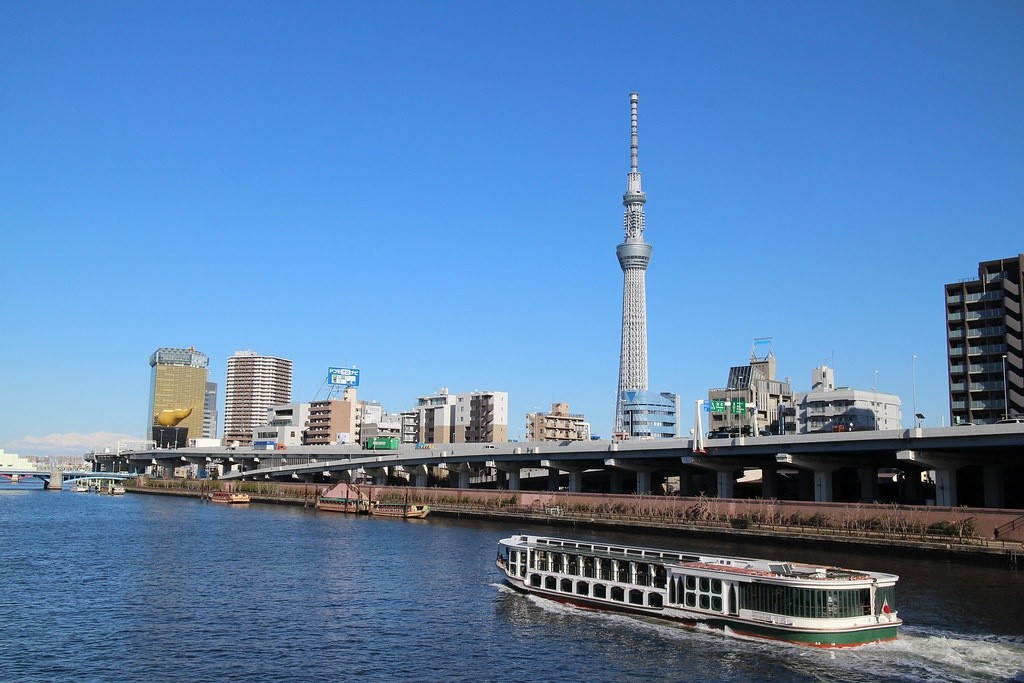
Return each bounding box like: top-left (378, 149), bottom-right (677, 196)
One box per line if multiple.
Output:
top-left (482, 445), bottom-right (495, 449)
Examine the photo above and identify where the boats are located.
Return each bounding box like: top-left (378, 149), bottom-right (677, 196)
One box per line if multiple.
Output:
top-left (211, 491), bottom-right (249, 503)
top-left (69, 486), bottom-right (86, 491)
top-left (108, 486), bottom-right (125, 495)
top-left (318, 497), bottom-right (369, 513)
top-left (371, 503), bottom-right (430, 519)
top-left (494, 532), bottom-right (903, 650)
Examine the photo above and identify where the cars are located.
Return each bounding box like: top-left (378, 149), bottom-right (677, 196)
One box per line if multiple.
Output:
top-left (993, 419), bottom-right (1024, 423)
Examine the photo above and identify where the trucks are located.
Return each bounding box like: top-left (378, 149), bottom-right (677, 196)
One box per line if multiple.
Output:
top-left (254, 441), bottom-right (286, 450)
top-left (362, 437), bottom-right (399, 451)
top-left (415, 442), bottom-right (436, 449)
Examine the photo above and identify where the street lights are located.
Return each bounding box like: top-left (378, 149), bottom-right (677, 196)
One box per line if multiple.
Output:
top-left (175, 428), bottom-right (179, 450)
top-left (727, 386), bottom-right (735, 427)
top-left (1002, 355), bottom-right (1008, 419)
top-left (874, 370), bottom-right (879, 430)
top-left (160, 429), bottom-right (165, 448)
top-left (738, 376), bottom-right (746, 436)
top-left (911, 355), bottom-right (918, 427)
